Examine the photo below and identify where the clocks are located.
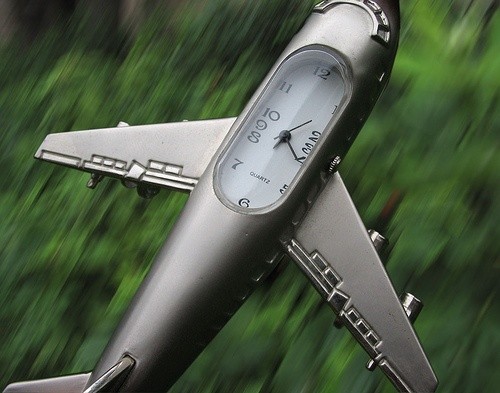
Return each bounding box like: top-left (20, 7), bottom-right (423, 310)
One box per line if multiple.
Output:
top-left (214, 44), bottom-right (355, 213)
